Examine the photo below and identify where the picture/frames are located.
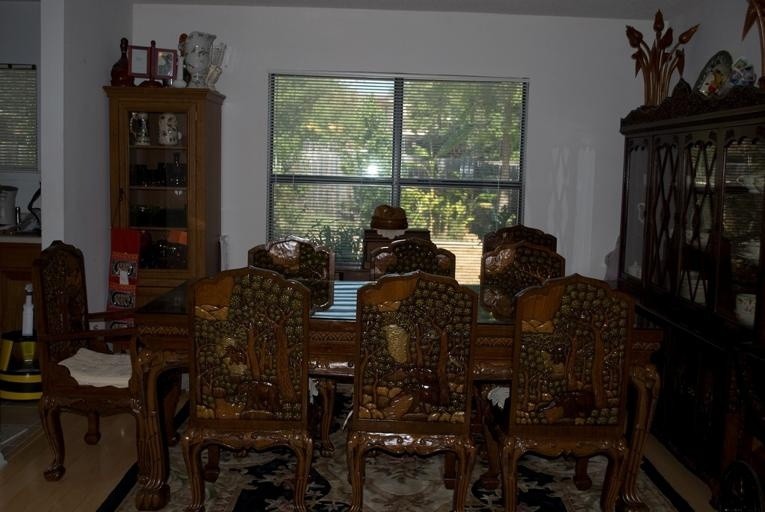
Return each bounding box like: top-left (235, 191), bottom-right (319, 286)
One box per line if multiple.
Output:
top-left (126, 44), bottom-right (177, 81)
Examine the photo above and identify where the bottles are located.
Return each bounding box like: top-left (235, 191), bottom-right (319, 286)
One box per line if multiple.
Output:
top-left (128, 112), bottom-right (150, 147)
top-left (157, 113), bottom-right (179, 146)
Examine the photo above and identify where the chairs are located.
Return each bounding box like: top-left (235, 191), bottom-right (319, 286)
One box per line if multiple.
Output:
top-left (247, 235), bottom-right (337, 280)
top-left (186, 264), bottom-right (317, 511)
top-left (344, 267), bottom-right (484, 512)
top-left (478, 244), bottom-right (567, 280)
top-left (33, 237), bottom-right (184, 490)
top-left (482, 226), bottom-right (559, 254)
top-left (367, 237), bottom-right (458, 280)
top-left (484, 277), bottom-right (641, 512)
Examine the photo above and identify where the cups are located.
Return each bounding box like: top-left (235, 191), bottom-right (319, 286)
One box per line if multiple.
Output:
top-left (736, 175), bottom-right (765, 193)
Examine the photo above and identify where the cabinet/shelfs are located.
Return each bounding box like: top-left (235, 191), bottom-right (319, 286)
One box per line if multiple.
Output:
top-left (0, 242), bottom-right (42, 338)
top-left (616, 80), bottom-right (765, 512)
top-left (102, 83), bottom-right (226, 371)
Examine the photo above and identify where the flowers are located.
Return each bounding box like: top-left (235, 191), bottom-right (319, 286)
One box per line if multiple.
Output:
top-left (625, 8), bottom-right (702, 103)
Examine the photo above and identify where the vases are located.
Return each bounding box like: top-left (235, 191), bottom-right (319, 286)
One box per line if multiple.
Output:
top-left (179, 31), bottom-right (217, 89)
top-left (641, 66), bottom-right (673, 107)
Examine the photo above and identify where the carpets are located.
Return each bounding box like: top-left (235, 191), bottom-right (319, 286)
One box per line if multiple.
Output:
top-left (97, 380), bottom-right (696, 512)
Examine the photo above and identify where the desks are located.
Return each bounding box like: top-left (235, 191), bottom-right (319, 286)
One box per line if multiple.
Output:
top-left (127, 280), bottom-right (666, 512)
